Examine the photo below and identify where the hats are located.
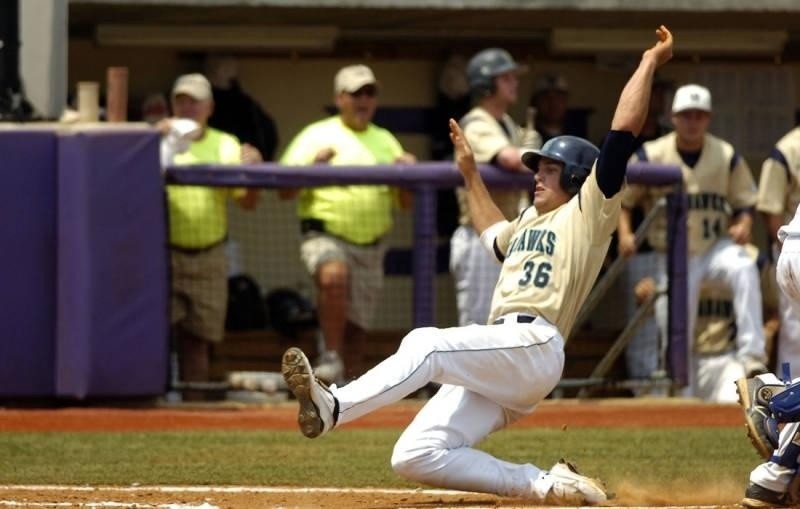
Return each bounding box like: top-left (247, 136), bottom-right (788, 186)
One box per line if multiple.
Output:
top-left (333, 65), bottom-right (377, 97)
top-left (672, 84), bottom-right (712, 117)
top-left (169, 73), bottom-right (213, 103)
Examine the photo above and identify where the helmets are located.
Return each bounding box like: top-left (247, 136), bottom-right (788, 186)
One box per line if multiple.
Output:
top-left (522, 136), bottom-right (601, 195)
top-left (465, 48), bottom-right (519, 96)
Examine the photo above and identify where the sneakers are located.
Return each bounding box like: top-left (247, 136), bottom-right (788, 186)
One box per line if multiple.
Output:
top-left (733, 356), bottom-right (800, 506)
top-left (544, 458), bottom-right (607, 508)
top-left (315, 349), bottom-right (346, 388)
top-left (280, 347), bottom-right (339, 440)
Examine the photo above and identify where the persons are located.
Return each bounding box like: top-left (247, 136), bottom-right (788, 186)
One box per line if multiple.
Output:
top-left (277, 63), bottom-right (419, 391)
top-left (617, 82), bottom-right (767, 402)
top-left (449, 47), bottom-right (544, 329)
top-left (732, 115), bottom-right (800, 509)
top-left (524, 69), bottom-right (570, 143)
top-left (138, 60), bottom-right (264, 405)
top-left (280, 26), bottom-right (674, 507)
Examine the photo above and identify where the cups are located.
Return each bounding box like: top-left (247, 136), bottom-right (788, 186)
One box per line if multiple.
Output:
top-left (76, 83), bottom-right (99, 122)
top-left (107, 65), bottom-right (129, 122)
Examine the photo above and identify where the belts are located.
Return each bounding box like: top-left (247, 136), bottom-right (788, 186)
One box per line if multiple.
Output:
top-left (490, 313), bottom-right (537, 324)
